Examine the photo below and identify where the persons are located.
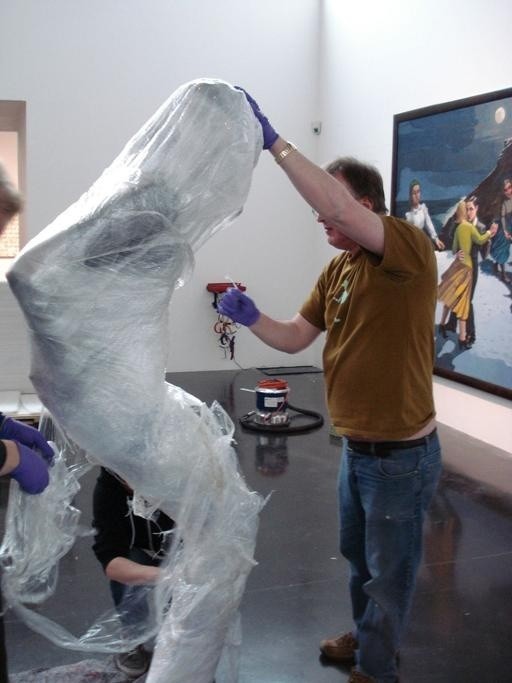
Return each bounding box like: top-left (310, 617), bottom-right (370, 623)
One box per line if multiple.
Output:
top-left (403, 179), bottom-right (445, 250)
top-left (437, 179), bottom-right (512, 350)
top-left (0, 168), bottom-right (53, 683)
top-left (92, 465), bottom-right (178, 676)
top-left (218, 85), bottom-right (443, 683)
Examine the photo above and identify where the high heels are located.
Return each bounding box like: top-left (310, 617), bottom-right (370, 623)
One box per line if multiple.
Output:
top-left (438, 321), bottom-right (456, 339)
top-left (458, 333), bottom-right (474, 350)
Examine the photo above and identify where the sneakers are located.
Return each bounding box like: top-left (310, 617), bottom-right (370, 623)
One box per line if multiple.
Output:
top-left (113, 646), bottom-right (149, 676)
top-left (347, 668), bottom-right (374, 683)
top-left (319, 632), bottom-right (358, 660)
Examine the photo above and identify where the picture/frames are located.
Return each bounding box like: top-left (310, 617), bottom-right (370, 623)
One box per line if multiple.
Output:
top-left (390, 88), bottom-right (512, 397)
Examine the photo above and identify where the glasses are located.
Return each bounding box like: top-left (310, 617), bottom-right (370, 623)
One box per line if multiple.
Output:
top-left (310, 207), bottom-right (319, 219)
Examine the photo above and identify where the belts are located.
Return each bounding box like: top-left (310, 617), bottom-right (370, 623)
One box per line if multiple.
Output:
top-left (348, 437), bottom-right (423, 457)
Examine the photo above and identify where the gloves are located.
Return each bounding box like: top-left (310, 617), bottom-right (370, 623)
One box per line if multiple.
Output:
top-left (233, 85), bottom-right (278, 150)
top-left (217, 287), bottom-right (260, 327)
top-left (0, 414), bottom-right (55, 493)
